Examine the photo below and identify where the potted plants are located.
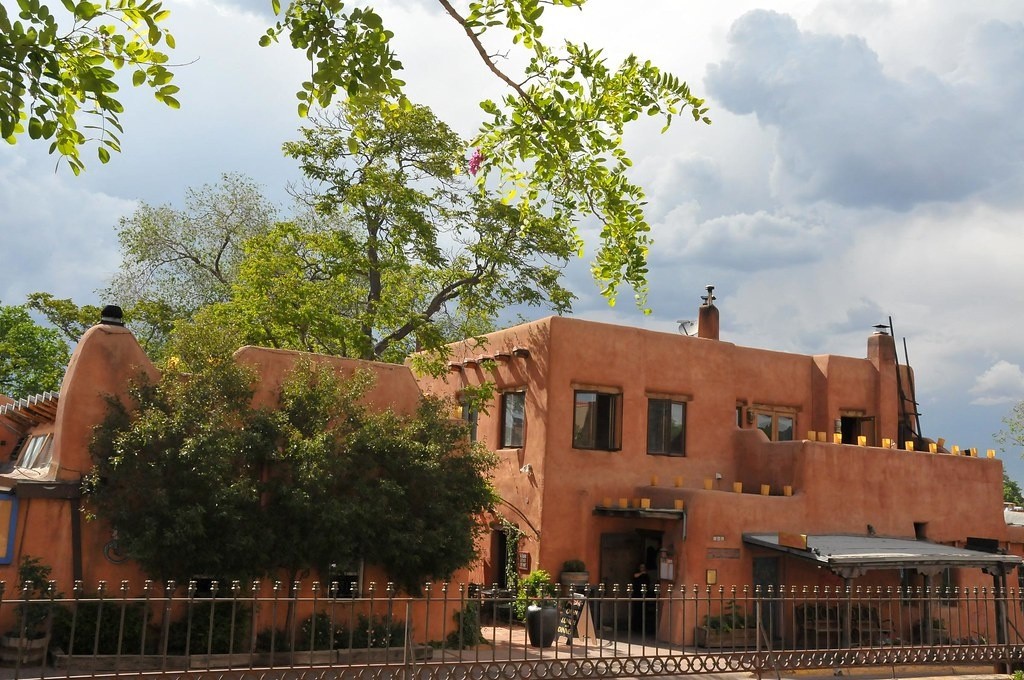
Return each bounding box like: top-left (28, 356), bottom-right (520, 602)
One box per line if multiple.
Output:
top-left (514, 570), bottom-right (561, 648)
top-left (2, 555), bottom-right (66, 668)
top-left (915, 616), bottom-right (948, 645)
top-left (560, 559), bottom-right (589, 598)
top-left (695, 600), bottom-right (771, 648)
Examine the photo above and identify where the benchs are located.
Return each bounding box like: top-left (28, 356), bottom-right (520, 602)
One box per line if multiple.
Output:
top-left (795, 604), bottom-right (842, 649)
top-left (852, 607), bottom-right (895, 648)
